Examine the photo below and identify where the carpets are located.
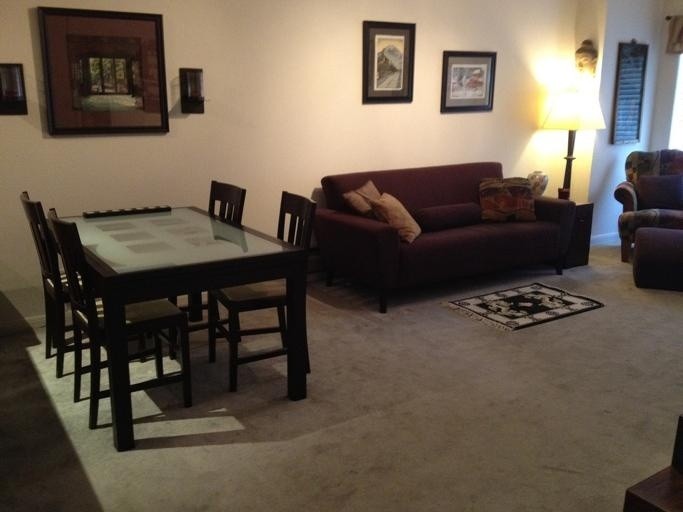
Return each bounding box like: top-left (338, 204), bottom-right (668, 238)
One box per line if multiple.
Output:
top-left (440, 281), bottom-right (603, 332)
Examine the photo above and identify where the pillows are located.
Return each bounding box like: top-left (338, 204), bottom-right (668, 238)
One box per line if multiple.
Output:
top-left (341, 178), bottom-right (383, 217)
top-left (617, 207), bottom-right (683, 251)
top-left (411, 201), bottom-right (480, 231)
top-left (634, 174), bottom-right (683, 210)
top-left (369, 190), bottom-right (422, 245)
top-left (476, 177), bottom-right (535, 224)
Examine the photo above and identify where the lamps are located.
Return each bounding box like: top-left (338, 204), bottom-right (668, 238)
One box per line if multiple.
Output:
top-left (0, 62), bottom-right (28, 116)
top-left (539, 68), bottom-right (605, 202)
top-left (179, 68), bottom-right (205, 114)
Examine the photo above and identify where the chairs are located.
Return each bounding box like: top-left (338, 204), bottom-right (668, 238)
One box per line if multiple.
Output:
top-left (148, 179), bottom-right (246, 365)
top-left (632, 226), bottom-right (683, 291)
top-left (614, 150), bottom-right (683, 264)
top-left (206, 190), bottom-right (312, 393)
top-left (20, 190), bottom-right (147, 381)
top-left (47, 207), bottom-right (193, 430)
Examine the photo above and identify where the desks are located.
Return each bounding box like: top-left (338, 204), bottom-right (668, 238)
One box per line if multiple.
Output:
top-left (569, 197), bottom-right (594, 269)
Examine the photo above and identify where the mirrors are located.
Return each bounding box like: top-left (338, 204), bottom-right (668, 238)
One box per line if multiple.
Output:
top-left (610, 40), bottom-right (649, 146)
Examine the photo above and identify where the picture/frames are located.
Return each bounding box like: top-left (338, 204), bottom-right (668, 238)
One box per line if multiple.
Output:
top-left (362, 20), bottom-right (415, 104)
top-left (36, 7), bottom-right (170, 136)
top-left (439, 51), bottom-right (496, 114)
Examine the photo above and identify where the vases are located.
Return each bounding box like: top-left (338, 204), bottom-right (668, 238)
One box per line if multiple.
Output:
top-left (528, 170), bottom-right (548, 199)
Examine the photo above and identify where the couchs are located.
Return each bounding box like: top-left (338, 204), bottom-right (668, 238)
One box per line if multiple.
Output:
top-left (315, 161), bottom-right (575, 314)
